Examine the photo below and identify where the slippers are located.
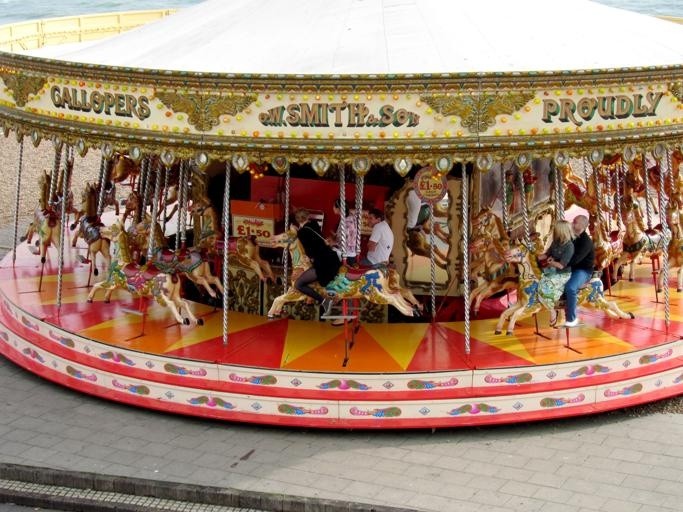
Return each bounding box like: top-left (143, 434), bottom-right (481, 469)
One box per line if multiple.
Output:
top-left (550, 310), bottom-right (558, 326)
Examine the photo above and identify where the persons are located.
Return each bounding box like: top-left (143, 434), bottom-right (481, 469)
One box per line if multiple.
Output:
top-left (562, 215), bottom-right (595, 328)
top-left (524, 166), bottom-right (534, 214)
top-left (330, 198), bottom-right (357, 267)
top-left (359, 208), bottom-right (394, 268)
top-left (293, 208), bottom-right (341, 316)
top-left (506, 170), bottom-right (516, 217)
top-left (536, 221), bottom-right (576, 327)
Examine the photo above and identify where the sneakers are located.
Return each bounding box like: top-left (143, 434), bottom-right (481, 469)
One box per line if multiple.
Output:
top-left (565, 318), bottom-right (578, 327)
top-left (323, 299), bottom-right (333, 316)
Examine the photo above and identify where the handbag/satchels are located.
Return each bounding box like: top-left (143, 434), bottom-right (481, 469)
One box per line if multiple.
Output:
top-left (332, 246), bottom-right (343, 263)
